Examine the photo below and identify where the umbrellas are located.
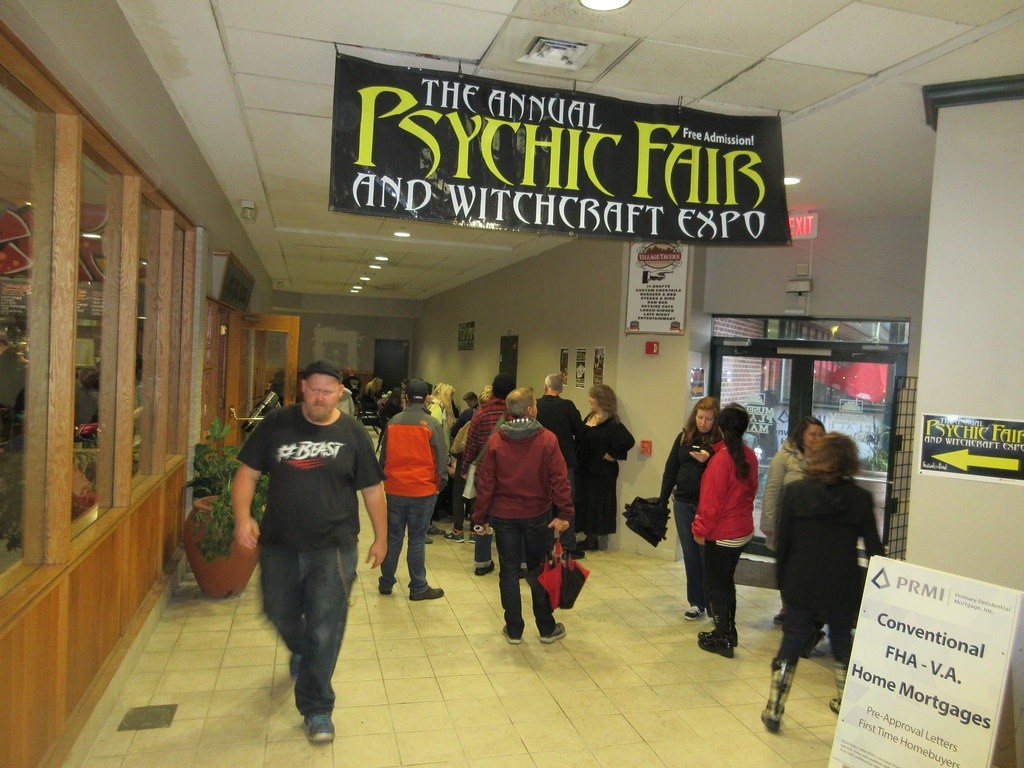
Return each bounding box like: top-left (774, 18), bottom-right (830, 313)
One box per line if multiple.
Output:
top-left (621, 497), bottom-right (671, 548)
top-left (825, 360), bottom-right (889, 429)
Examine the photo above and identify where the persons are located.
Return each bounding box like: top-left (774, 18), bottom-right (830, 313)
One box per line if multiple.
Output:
top-left (761, 431), bottom-right (893, 729)
top-left (761, 417), bottom-right (827, 625)
top-left (461, 372), bottom-right (528, 580)
top-left (379, 379), bottom-right (448, 601)
top-left (574, 384), bottom-right (635, 549)
top-left (658, 399), bottom-right (724, 621)
top-left (1, 335), bottom-right (26, 446)
top-left (534, 373), bottom-right (582, 559)
top-left (474, 387), bottom-right (574, 644)
top-left (691, 402), bottom-right (758, 658)
top-left (230, 360), bottom-right (388, 744)
top-left (425, 382), bottom-right (493, 544)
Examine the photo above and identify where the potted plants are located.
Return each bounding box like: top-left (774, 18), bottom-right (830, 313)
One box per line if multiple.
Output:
top-left (182, 417), bottom-right (270, 600)
top-left (854, 415), bottom-right (892, 508)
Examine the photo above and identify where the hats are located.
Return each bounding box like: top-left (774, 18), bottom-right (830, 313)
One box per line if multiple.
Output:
top-left (407, 378), bottom-right (427, 400)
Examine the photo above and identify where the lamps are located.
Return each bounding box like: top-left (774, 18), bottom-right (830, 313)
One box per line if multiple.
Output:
top-left (241, 201), bottom-right (256, 223)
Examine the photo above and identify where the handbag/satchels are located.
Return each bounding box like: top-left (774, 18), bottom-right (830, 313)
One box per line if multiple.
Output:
top-left (462, 463), bottom-right (476, 500)
top-left (450, 420), bottom-right (471, 454)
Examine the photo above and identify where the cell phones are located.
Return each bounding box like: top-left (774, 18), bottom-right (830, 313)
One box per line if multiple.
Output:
top-left (692, 446), bottom-right (700, 452)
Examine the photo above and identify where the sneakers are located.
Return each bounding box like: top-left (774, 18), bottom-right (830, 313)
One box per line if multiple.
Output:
top-left (410, 586), bottom-right (444, 600)
top-left (469, 532), bottom-right (476, 544)
top-left (444, 530), bottom-right (465, 542)
top-left (540, 622), bottom-right (566, 643)
top-left (305, 710), bottom-right (335, 741)
top-left (380, 585), bottom-right (392, 594)
top-left (290, 652), bottom-right (301, 677)
top-left (502, 624), bottom-right (522, 643)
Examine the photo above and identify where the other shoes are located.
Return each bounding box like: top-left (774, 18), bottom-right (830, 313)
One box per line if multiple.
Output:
top-left (562, 550), bottom-right (586, 559)
top-left (475, 560), bottom-right (494, 575)
top-left (427, 525), bottom-right (446, 535)
top-left (425, 537), bottom-right (432, 543)
top-left (576, 536), bottom-right (598, 550)
top-left (684, 605), bottom-right (704, 621)
top-left (518, 568), bottom-right (528, 578)
top-left (773, 607), bottom-right (785, 624)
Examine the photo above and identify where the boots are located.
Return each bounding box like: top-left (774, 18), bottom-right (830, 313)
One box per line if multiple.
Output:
top-left (698, 602), bottom-right (737, 657)
top-left (829, 659), bottom-right (849, 714)
top-left (761, 658), bottom-right (796, 730)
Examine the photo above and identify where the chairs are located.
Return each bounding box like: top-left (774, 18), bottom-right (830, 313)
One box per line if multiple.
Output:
top-left (354, 395), bottom-right (392, 455)
